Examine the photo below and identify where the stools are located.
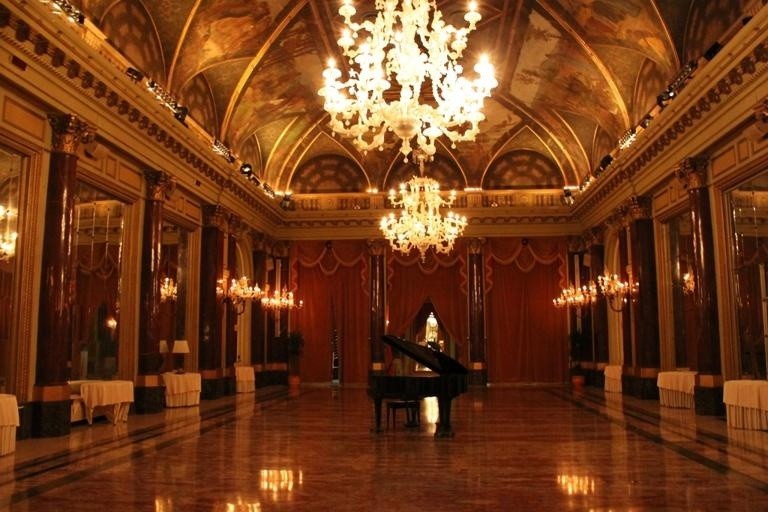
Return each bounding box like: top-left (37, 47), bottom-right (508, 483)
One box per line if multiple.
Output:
top-left (387, 399), bottom-right (420, 430)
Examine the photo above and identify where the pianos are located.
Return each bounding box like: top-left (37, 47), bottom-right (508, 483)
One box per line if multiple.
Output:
top-left (366, 335), bottom-right (468, 437)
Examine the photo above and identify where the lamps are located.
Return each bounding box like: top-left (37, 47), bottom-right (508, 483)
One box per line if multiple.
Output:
top-left (597, 272), bottom-right (629, 312)
top-left (552, 284), bottom-right (585, 319)
top-left (380, 176), bottom-right (469, 265)
top-left (173, 340), bottom-right (190, 374)
top-left (159, 276), bottom-right (179, 304)
top-left (316, 0), bottom-right (500, 166)
top-left (271, 286), bottom-right (303, 311)
top-left (227, 276), bottom-right (262, 315)
top-left (0, 150), bottom-right (23, 261)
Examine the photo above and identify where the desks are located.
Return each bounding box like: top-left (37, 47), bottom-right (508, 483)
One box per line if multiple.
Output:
top-left (81, 380), bottom-right (134, 424)
top-left (162, 370), bottom-right (202, 407)
top-left (657, 370), bottom-right (698, 409)
top-left (0, 393), bottom-right (18, 453)
top-left (723, 379), bottom-right (768, 430)
top-left (604, 365), bottom-right (623, 392)
top-left (235, 367), bottom-right (256, 392)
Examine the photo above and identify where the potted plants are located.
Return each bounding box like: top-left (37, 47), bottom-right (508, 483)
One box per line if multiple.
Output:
top-left (569, 329), bottom-right (585, 386)
top-left (288, 330), bottom-right (304, 387)
